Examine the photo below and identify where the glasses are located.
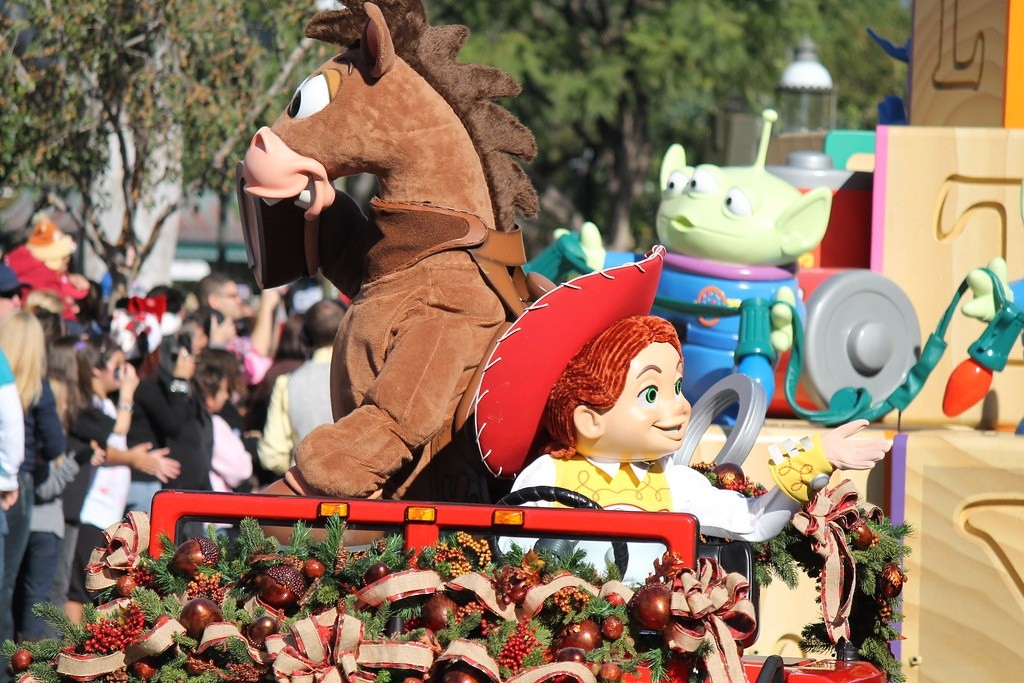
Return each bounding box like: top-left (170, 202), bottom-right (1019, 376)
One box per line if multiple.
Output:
top-left (0, 287), bottom-right (24, 299)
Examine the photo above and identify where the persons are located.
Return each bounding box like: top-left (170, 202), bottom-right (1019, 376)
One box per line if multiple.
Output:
top-left (0, 217), bottom-right (349, 683)
top-left (474, 246), bottom-right (893, 587)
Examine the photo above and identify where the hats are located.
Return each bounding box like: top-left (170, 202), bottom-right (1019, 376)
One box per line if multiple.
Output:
top-left (26, 215), bottom-right (77, 263)
top-left (0, 262), bottom-right (33, 291)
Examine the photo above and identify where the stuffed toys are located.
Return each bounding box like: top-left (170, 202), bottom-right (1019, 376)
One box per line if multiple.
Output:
top-left (242, 0), bottom-right (540, 498)
